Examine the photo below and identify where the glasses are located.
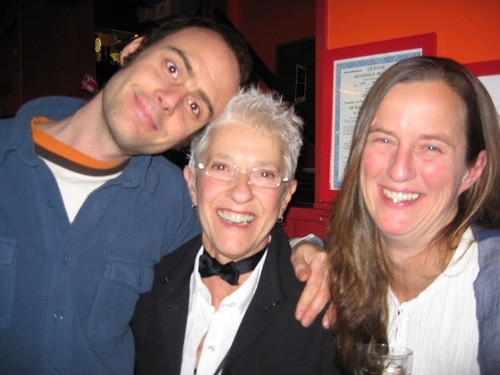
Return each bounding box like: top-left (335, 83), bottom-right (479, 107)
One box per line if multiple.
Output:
top-left (194, 157), bottom-right (288, 188)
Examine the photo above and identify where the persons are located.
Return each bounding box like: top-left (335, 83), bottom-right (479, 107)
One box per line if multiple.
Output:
top-left (325, 56), bottom-right (500, 375)
top-left (0, 18), bottom-right (342, 375)
top-left (116, 81), bottom-right (339, 375)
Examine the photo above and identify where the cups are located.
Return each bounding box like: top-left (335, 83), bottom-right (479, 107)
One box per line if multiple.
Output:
top-left (355, 344), bottom-right (414, 375)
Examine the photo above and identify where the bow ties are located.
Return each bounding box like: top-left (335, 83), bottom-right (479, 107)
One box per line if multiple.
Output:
top-left (198, 246), bottom-right (267, 286)
